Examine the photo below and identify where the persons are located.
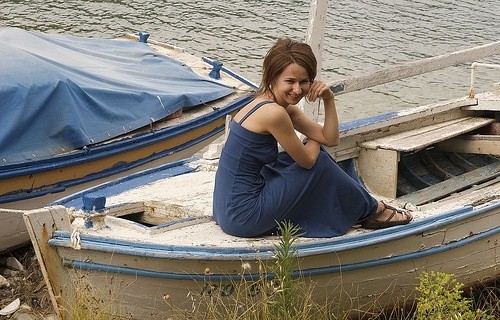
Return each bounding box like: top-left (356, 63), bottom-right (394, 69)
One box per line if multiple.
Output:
top-left (212, 40), bottom-right (414, 240)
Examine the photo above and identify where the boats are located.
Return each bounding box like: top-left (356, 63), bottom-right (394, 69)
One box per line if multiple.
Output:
top-left (0, 34), bottom-right (267, 263)
top-left (16, 93), bottom-right (500, 320)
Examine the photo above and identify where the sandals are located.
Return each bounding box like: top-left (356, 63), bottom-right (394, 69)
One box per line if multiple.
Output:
top-left (363, 200), bottom-right (414, 229)
